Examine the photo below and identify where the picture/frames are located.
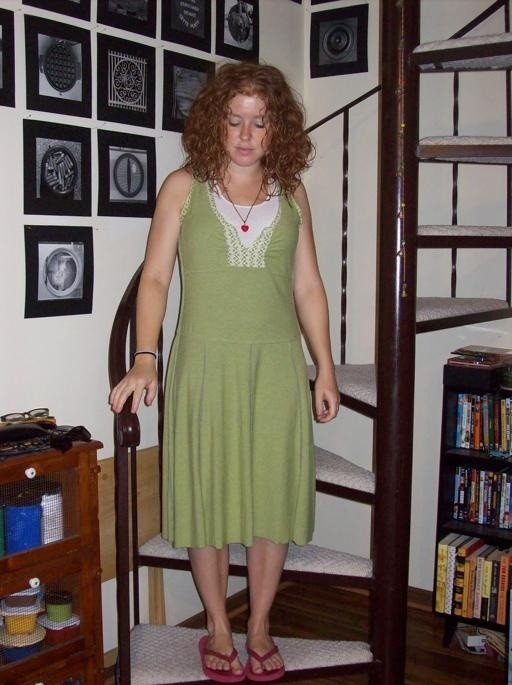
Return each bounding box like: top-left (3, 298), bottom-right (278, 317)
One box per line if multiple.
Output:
top-left (216, 0), bottom-right (259, 64)
top-left (96, 33), bottom-right (156, 128)
top-left (24, 226), bottom-right (94, 318)
top-left (22, 0), bottom-right (91, 21)
top-left (161, 0), bottom-right (211, 53)
top-left (162, 49), bottom-right (216, 133)
top-left (310, 4), bottom-right (369, 79)
top-left (97, 0), bottom-right (157, 39)
top-left (24, 14), bottom-right (92, 118)
top-left (23, 119), bottom-right (91, 217)
top-left (98, 129), bottom-right (156, 217)
top-left (1, 8), bottom-right (15, 108)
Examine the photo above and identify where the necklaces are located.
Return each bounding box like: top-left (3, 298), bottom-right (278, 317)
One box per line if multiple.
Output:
top-left (213, 168), bottom-right (268, 232)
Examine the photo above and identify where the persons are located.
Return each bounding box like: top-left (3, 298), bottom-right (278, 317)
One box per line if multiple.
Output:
top-left (109, 63), bottom-right (340, 684)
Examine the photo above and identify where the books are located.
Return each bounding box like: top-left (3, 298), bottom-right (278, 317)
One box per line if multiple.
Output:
top-left (434, 393), bottom-right (512, 626)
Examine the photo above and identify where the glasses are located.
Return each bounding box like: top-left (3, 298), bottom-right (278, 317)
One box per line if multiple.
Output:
top-left (0, 408), bottom-right (49, 422)
top-left (49, 426), bottom-right (92, 453)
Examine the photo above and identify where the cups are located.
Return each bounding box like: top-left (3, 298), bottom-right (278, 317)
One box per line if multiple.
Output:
top-left (47, 605), bottom-right (73, 620)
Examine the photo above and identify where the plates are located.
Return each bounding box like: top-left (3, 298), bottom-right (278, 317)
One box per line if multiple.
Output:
top-left (0, 623), bottom-right (46, 648)
top-left (38, 614), bottom-right (79, 630)
top-left (41, 146), bottom-right (77, 195)
top-left (44, 249), bottom-right (80, 295)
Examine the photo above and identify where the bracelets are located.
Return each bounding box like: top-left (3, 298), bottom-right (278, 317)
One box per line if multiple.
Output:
top-left (133, 352), bottom-right (156, 359)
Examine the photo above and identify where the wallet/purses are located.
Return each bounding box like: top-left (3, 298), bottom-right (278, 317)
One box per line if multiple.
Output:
top-left (0, 425), bottom-right (45, 441)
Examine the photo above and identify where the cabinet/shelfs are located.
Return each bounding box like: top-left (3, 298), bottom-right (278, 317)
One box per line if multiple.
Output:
top-left (432, 364), bottom-right (511, 681)
top-left (0, 439), bottom-right (103, 685)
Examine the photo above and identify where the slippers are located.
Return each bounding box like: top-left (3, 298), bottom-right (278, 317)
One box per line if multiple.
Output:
top-left (199, 635), bottom-right (246, 683)
top-left (246, 645), bottom-right (285, 682)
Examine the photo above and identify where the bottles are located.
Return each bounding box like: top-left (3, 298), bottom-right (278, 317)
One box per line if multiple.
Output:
top-left (4, 491), bottom-right (42, 554)
top-left (40, 482), bottom-right (64, 544)
